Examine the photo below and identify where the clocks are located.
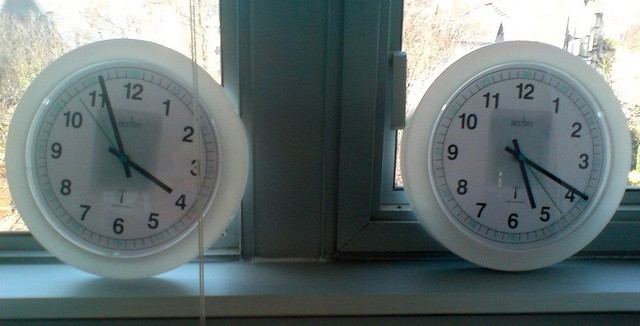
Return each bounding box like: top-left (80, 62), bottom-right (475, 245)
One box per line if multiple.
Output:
top-left (4, 38), bottom-right (248, 282)
top-left (401, 40), bottom-right (631, 272)
